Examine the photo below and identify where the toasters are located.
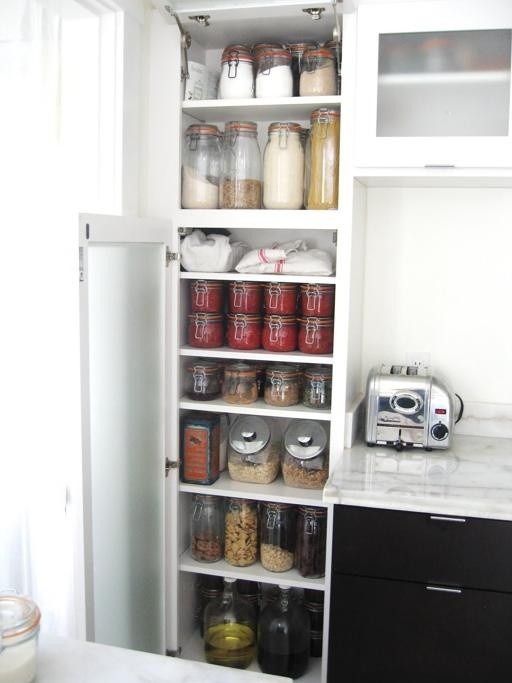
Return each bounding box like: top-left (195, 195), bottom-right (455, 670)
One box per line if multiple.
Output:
top-left (360, 360), bottom-right (464, 451)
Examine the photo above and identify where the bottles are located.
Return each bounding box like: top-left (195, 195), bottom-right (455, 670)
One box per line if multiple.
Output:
top-left (262, 315), bottom-right (298, 352)
top-left (229, 281), bottom-right (262, 314)
top-left (203, 577), bottom-right (258, 670)
top-left (263, 122), bottom-right (304, 209)
top-left (305, 109), bottom-right (340, 210)
top-left (219, 121), bottom-right (263, 209)
top-left (286, 43), bottom-right (318, 98)
top-left (219, 53), bottom-right (254, 100)
top-left (182, 125), bottom-right (223, 209)
top-left (185, 362), bottom-right (221, 400)
top-left (255, 53), bottom-right (295, 97)
top-left (200, 587), bottom-right (223, 638)
top-left (226, 414), bottom-right (283, 484)
top-left (259, 503), bottom-right (297, 572)
top-left (190, 495), bottom-right (223, 563)
top-left (237, 594), bottom-right (259, 648)
top-left (256, 585), bottom-right (311, 679)
top-left (325, 42), bottom-right (341, 94)
top-left (220, 363), bottom-right (259, 404)
top-left (297, 316), bottom-right (334, 354)
top-left (224, 498), bottom-right (259, 567)
top-left (280, 421), bottom-right (330, 490)
top-left (264, 283), bottom-right (299, 315)
top-left (227, 313), bottom-right (263, 350)
top-left (263, 365), bottom-right (300, 405)
top-left (253, 43), bottom-right (283, 74)
top-left (299, 284), bottom-right (335, 317)
top-left (223, 44), bottom-right (250, 55)
top-left (295, 506), bottom-right (327, 580)
top-left (299, 51), bottom-right (338, 96)
top-left (187, 312), bottom-right (225, 347)
top-left (190, 279), bottom-right (224, 313)
top-left (303, 366), bottom-right (332, 410)
top-left (305, 602), bottom-right (323, 657)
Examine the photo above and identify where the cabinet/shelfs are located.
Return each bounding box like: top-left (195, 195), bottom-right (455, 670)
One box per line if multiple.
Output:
top-left (353, 1), bottom-right (512, 188)
top-left (321, 433), bottom-right (512, 683)
top-left (78, 0), bottom-right (363, 681)
